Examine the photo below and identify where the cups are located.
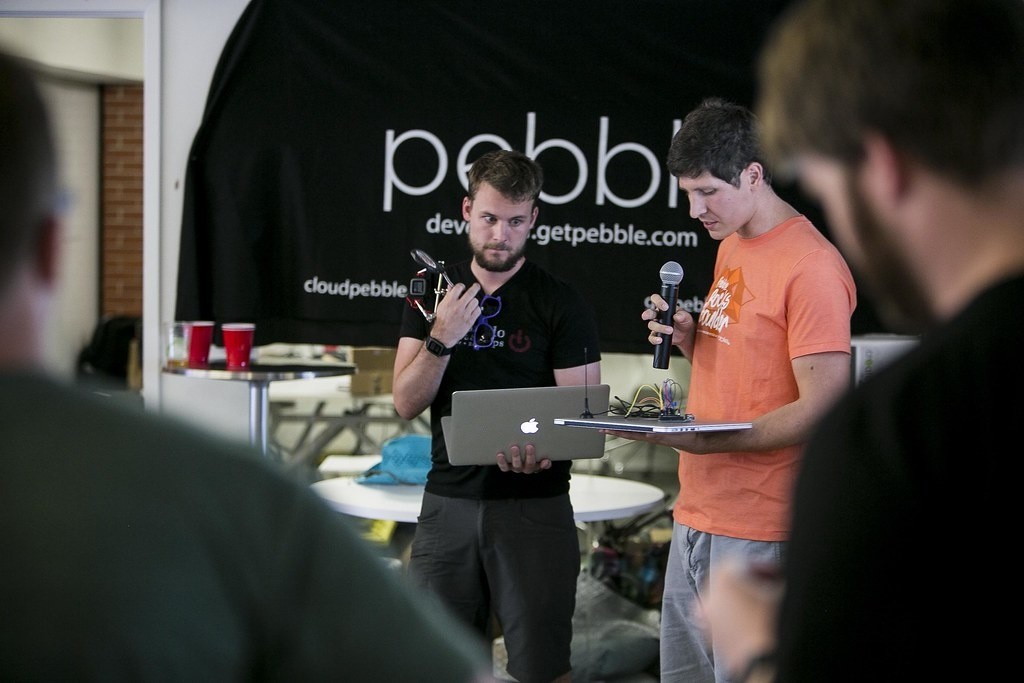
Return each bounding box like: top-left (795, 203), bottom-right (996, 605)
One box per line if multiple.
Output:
top-left (162, 321), bottom-right (193, 368)
top-left (182, 320), bottom-right (215, 369)
top-left (221, 323), bottom-right (255, 371)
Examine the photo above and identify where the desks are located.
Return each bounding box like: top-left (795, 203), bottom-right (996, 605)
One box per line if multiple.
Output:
top-left (310, 469), bottom-right (665, 522)
top-left (266, 375), bottom-right (433, 471)
top-left (162, 360), bottom-right (356, 458)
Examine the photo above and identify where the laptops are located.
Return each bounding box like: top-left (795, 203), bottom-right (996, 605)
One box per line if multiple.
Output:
top-left (441, 385), bottom-right (611, 466)
top-left (553, 416), bottom-right (753, 434)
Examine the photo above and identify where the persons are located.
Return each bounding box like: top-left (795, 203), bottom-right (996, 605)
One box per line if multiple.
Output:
top-left (641, 100), bottom-right (857, 683)
top-left (389, 152), bottom-right (603, 683)
top-left (0, 48), bottom-right (515, 683)
top-left (683, 0), bottom-right (1024, 683)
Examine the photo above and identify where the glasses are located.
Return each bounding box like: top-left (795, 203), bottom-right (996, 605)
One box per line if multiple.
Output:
top-left (473, 295), bottom-right (501, 351)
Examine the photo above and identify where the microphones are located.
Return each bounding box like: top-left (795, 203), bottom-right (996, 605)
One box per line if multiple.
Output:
top-left (652, 261), bottom-right (684, 369)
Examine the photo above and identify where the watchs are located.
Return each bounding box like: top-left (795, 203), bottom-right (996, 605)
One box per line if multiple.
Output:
top-left (426, 331), bottom-right (453, 357)
